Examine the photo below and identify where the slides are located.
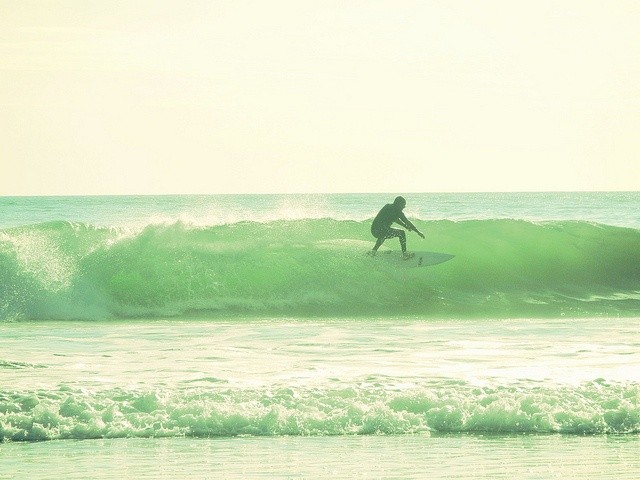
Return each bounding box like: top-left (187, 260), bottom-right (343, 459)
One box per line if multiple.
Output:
top-left (363, 250), bottom-right (456, 267)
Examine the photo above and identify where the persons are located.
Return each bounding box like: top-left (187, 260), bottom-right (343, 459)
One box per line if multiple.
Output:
top-left (371, 196), bottom-right (426, 260)
